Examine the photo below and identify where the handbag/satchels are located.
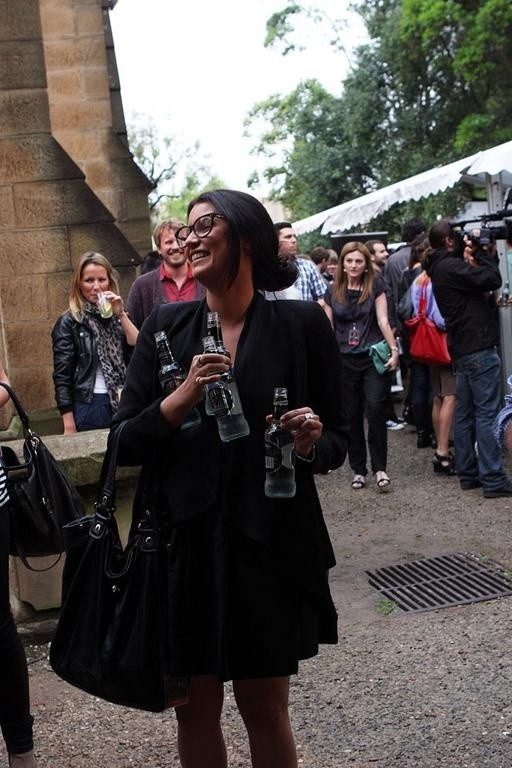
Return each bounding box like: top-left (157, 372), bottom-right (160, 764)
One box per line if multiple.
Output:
top-left (0, 432), bottom-right (86, 557)
top-left (404, 316), bottom-right (451, 366)
top-left (49, 514), bottom-right (194, 712)
top-left (369, 339), bottom-right (392, 374)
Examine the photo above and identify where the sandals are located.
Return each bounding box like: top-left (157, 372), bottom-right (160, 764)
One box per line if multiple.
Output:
top-left (352, 474), bottom-right (365, 488)
top-left (376, 471), bottom-right (390, 487)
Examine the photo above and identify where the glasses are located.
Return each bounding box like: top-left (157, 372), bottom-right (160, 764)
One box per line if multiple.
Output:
top-left (175, 212), bottom-right (225, 248)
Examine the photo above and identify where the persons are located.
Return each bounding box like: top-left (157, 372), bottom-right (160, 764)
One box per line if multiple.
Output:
top-left (0, 374), bottom-right (39, 767)
top-left (127, 215), bottom-right (510, 499)
top-left (50, 245), bottom-right (140, 439)
top-left (105, 187), bottom-right (354, 767)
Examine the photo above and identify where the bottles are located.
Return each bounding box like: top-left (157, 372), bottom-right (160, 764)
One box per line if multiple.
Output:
top-left (149, 329), bottom-right (202, 428)
top-left (262, 387), bottom-right (298, 495)
top-left (196, 335), bottom-right (253, 442)
top-left (203, 311), bottom-right (226, 415)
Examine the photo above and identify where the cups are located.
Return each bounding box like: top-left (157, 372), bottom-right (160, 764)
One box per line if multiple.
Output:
top-left (92, 290), bottom-right (115, 318)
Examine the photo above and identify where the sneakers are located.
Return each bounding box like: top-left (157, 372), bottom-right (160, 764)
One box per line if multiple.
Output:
top-left (386, 417), bottom-right (408, 430)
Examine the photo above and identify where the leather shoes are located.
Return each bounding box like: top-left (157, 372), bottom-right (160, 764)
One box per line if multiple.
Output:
top-left (417, 430), bottom-right (457, 474)
top-left (460, 477), bottom-right (512, 498)
